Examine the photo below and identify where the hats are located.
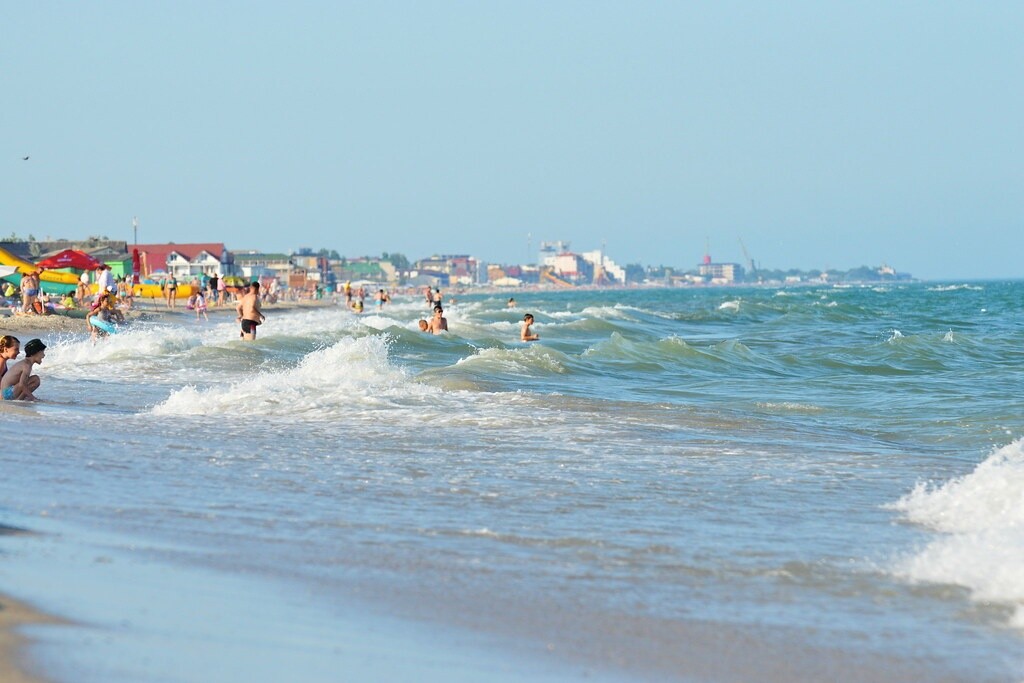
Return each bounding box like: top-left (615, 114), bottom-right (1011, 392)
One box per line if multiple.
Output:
top-left (25, 339), bottom-right (46, 357)
top-left (169, 271), bottom-right (172, 275)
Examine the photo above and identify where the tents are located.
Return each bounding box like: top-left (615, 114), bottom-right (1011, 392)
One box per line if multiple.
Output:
top-left (35, 249), bottom-right (112, 271)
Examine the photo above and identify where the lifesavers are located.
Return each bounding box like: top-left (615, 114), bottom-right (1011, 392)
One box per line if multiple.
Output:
top-left (90, 316), bottom-right (117, 335)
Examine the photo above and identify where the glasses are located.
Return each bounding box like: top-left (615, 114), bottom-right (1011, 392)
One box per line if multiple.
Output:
top-left (438, 310), bottom-right (443, 313)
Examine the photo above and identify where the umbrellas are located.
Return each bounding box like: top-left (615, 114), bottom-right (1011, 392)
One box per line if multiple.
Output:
top-left (0, 265), bottom-right (19, 278)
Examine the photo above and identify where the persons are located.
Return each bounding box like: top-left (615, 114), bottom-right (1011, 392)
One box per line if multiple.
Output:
top-left (352, 288), bottom-right (364, 312)
top-left (425, 286), bottom-right (433, 307)
top-left (165, 271), bottom-right (177, 309)
top-left (433, 290), bottom-right (442, 307)
top-left (263, 277), bottom-right (332, 304)
top-left (1, 338), bottom-right (46, 401)
top-left (0, 336), bottom-right (21, 378)
top-left (450, 298), bottom-right (456, 304)
top-left (195, 292), bottom-right (209, 321)
top-left (508, 297), bottom-right (516, 306)
top-left (373, 289), bottom-right (391, 310)
top-left (236, 281), bottom-right (265, 340)
top-left (343, 279), bottom-right (353, 307)
top-left (2, 267), bottom-right (76, 318)
top-left (521, 314), bottom-right (539, 342)
top-left (426, 305), bottom-right (448, 334)
top-left (188, 273), bottom-right (230, 308)
top-left (419, 320), bottom-right (428, 331)
top-left (77, 265), bottom-right (137, 338)
